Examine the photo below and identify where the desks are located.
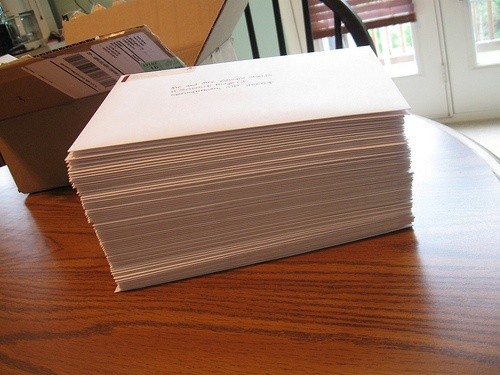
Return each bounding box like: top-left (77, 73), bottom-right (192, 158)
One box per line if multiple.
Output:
top-left (1, 113), bottom-right (498, 373)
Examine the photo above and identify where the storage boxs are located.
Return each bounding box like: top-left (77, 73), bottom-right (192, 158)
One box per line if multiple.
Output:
top-left (1, 0), bottom-right (250, 197)
